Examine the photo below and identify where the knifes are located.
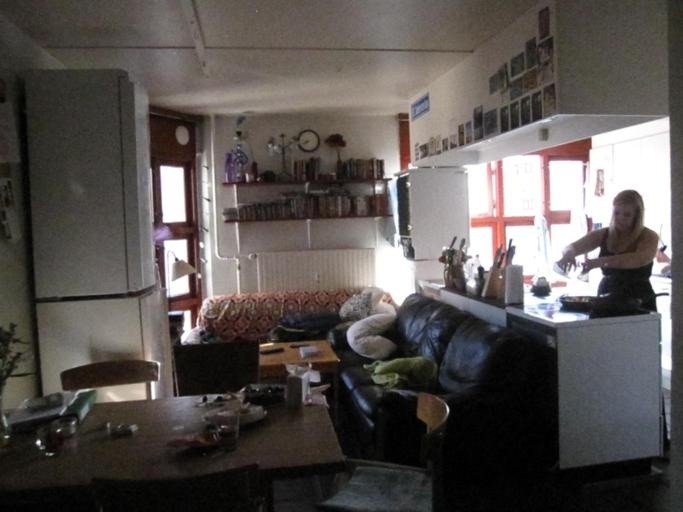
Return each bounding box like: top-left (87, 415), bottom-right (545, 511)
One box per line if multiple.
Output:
top-left (492, 245), bottom-right (505, 267)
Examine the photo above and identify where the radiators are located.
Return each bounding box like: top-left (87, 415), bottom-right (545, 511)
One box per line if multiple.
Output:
top-left (248, 247), bottom-right (377, 294)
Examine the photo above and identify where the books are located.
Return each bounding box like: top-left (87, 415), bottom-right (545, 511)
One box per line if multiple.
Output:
top-left (222, 156), bottom-right (389, 221)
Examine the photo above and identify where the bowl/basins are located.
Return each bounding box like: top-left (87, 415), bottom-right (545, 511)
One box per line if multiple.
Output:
top-left (226, 382), bottom-right (288, 405)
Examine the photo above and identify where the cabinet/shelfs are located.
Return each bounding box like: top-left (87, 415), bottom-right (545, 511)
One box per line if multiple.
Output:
top-left (390, 165), bottom-right (470, 262)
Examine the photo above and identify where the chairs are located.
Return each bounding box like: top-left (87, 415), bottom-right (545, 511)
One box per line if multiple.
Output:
top-left (170, 335), bottom-right (278, 511)
top-left (310, 390), bottom-right (453, 512)
top-left (59, 357), bottom-right (161, 402)
top-left (0, 483), bottom-right (106, 512)
top-left (94, 459), bottom-right (261, 511)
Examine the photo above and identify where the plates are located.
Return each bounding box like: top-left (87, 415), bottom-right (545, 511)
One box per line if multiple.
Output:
top-left (201, 405), bottom-right (266, 425)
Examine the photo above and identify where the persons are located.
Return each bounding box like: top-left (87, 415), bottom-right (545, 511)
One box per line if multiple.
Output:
top-left (554, 189), bottom-right (672, 476)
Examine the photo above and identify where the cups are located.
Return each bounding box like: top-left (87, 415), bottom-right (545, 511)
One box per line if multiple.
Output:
top-left (216, 416), bottom-right (240, 451)
top-left (36, 425), bottom-right (63, 457)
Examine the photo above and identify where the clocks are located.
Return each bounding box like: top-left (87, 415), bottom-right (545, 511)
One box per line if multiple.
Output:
top-left (297, 128), bottom-right (320, 154)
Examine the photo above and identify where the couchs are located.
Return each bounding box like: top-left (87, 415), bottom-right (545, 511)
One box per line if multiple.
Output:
top-left (328, 287), bottom-right (554, 505)
top-left (184, 284), bottom-right (399, 345)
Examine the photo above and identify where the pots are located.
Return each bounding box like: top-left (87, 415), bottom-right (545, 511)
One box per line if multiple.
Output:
top-left (559, 295), bottom-right (593, 309)
top-left (589, 287), bottom-right (669, 313)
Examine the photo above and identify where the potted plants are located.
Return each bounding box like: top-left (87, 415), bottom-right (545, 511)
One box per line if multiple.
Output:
top-left (0, 321), bottom-right (40, 445)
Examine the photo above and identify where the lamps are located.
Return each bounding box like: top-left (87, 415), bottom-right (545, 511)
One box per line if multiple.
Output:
top-left (166, 247), bottom-right (197, 331)
